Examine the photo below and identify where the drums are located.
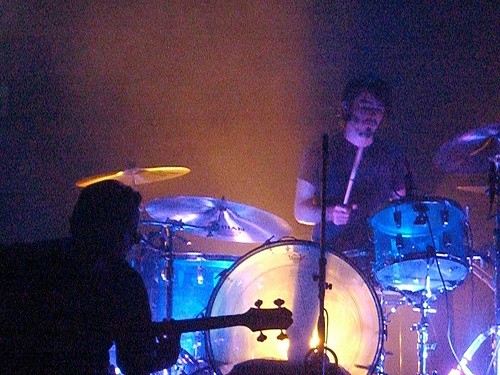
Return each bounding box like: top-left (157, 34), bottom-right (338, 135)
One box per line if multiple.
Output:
top-left (341, 248), bottom-right (384, 291)
top-left (367, 193), bottom-right (471, 296)
top-left (134, 229), bottom-right (261, 360)
top-left (109, 344), bottom-right (203, 375)
top-left (204, 240), bottom-right (387, 375)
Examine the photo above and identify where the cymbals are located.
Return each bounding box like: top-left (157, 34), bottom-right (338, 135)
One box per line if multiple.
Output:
top-left (433, 124), bottom-right (500, 175)
top-left (144, 196), bottom-right (292, 244)
top-left (75, 166), bottom-right (191, 188)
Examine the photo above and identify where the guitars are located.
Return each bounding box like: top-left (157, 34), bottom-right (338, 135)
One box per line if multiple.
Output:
top-left (107, 298), bottom-right (293, 350)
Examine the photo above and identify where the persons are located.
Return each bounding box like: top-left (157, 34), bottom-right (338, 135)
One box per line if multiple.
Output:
top-left (294, 72), bottom-right (408, 287)
top-left (0, 180), bottom-right (181, 375)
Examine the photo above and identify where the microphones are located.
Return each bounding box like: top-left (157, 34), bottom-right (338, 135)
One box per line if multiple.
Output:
top-left (321, 133), bottom-right (329, 160)
